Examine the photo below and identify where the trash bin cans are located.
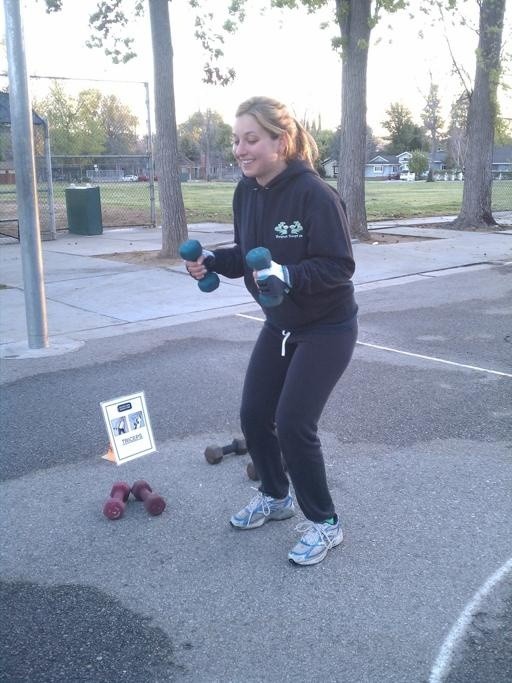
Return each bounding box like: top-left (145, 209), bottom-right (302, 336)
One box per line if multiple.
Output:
top-left (66, 186), bottom-right (103, 235)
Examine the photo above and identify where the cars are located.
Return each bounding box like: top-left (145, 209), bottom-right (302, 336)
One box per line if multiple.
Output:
top-left (120, 174), bottom-right (139, 181)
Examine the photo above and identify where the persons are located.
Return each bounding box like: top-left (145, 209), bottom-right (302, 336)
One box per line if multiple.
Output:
top-left (185, 97), bottom-right (359, 567)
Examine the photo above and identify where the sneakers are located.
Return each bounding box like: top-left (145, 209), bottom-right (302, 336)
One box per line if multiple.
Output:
top-left (229, 492), bottom-right (298, 529)
top-left (288, 514), bottom-right (343, 566)
